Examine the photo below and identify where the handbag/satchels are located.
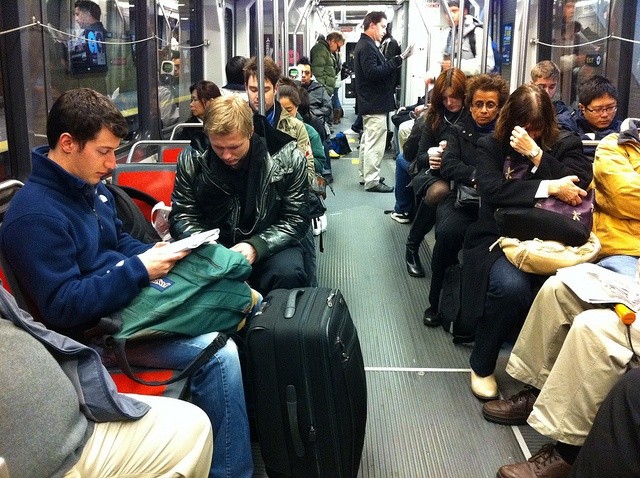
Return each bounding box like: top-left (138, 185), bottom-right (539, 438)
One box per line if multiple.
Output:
top-left (449, 179), bottom-right (482, 212)
top-left (493, 155), bottom-right (594, 245)
top-left (488, 233), bottom-right (601, 273)
top-left (329, 131), bottom-right (351, 155)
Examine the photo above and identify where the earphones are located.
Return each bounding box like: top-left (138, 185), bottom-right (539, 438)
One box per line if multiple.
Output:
top-left (274, 89), bottom-right (277, 93)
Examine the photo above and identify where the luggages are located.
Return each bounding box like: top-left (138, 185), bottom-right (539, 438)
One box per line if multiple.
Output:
top-left (244, 286), bottom-right (366, 478)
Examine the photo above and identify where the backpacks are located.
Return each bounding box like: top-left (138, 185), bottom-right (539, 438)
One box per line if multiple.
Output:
top-left (102, 180), bottom-right (161, 245)
top-left (470, 27), bottom-right (500, 74)
top-left (84, 241), bottom-right (252, 347)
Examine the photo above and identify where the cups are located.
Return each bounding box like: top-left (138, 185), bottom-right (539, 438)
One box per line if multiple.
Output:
top-left (428, 147), bottom-right (442, 170)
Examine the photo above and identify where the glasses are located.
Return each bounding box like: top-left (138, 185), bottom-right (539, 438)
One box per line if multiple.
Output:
top-left (470, 104), bottom-right (499, 109)
top-left (336, 41), bottom-right (340, 52)
top-left (585, 105), bottom-right (618, 115)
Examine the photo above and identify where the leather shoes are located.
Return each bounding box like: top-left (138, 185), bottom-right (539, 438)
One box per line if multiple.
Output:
top-left (482, 386), bottom-right (537, 425)
top-left (359, 177), bottom-right (385, 184)
top-left (496, 443), bottom-right (572, 478)
top-left (367, 180), bottom-right (394, 192)
top-left (470, 369), bottom-right (498, 399)
top-left (423, 307), bottom-right (441, 327)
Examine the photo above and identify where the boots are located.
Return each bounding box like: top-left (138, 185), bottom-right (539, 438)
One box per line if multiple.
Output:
top-left (406, 198), bottom-right (437, 277)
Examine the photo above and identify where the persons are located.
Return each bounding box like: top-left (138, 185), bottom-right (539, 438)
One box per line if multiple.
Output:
top-left (316, 35), bottom-right (342, 124)
top-left (71, 0), bottom-right (104, 67)
top-left (1, 277), bottom-right (214, 476)
top-left (392, 86), bottom-right (438, 157)
top-left (529, 60), bottom-right (579, 119)
top-left (557, 1), bottom-right (601, 71)
top-left (468, 84), bottom-right (593, 399)
top-left (220, 56), bottom-right (250, 91)
top-left (585, 117), bottom-right (640, 273)
top-left (389, 111), bottom-right (430, 224)
top-left (398, 103), bottom-right (434, 155)
top-left (240, 57), bottom-right (316, 290)
top-left (113, 52), bottom-right (180, 136)
top-left (276, 78), bottom-right (297, 94)
top-left (357, 25), bottom-right (399, 151)
top-left (1, 88), bottom-right (262, 478)
top-left (483, 261), bottom-right (640, 478)
top-left (294, 87), bottom-right (325, 145)
top-left (407, 68), bottom-right (468, 277)
top-left (353, 11), bottom-right (415, 193)
top-left (557, 76), bottom-right (628, 161)
top-left (425, 74), bottom-right (510, 327)
top-left (424, 0), bottom-right (501, 85)
top-left (310, 32), bottom-right (346, 159)
top-left (175, 81), bottom-right (221, 139)
top-left (570, 367), bottom-right (640, 478)
top-left (294, 57), bottom-right (333, 186)
top-left (170, 94), bottom-right (310, 297)
top-left (165, 51), bottom-right (180, 100)
top-left (274, 85), bottom-right (327, 177)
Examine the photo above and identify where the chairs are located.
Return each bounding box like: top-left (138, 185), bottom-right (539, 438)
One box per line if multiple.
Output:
top-left (157, 122), bottom-right (203, 164)
top-left (1, 178), bottom-right (189, 400)
top-left (111, 139), bottom-right (191, 224)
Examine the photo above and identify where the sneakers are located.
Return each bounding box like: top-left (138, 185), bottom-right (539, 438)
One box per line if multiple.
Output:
top-left (391, 210), bottom-right (410, 223)
